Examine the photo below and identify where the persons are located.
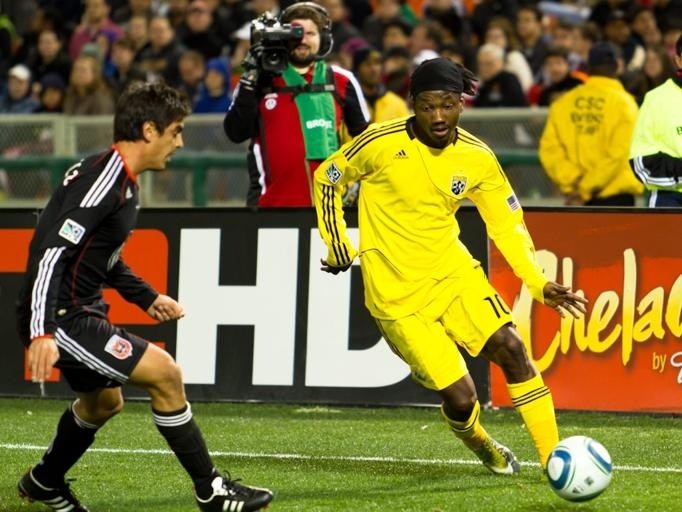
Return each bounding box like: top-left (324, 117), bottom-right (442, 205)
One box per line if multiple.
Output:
top-left (15, 81), bottom-right (274, 512)
top-left (313, 56), bottom-right (589, 476)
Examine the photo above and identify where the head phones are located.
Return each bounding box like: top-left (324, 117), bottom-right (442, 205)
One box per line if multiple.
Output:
top-left (277, 1), bottom-right (334, 60)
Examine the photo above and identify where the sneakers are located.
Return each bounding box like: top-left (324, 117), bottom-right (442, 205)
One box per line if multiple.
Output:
top-left (463, 425), bottom-right (520, 478)
top-left (194, 478), bottom-right (274, 512)
top-left (17, 467), bottom-right (88, 512)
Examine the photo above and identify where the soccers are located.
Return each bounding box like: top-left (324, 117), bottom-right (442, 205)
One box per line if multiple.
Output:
top-left (546, 436), bottom-right (612, 500)
